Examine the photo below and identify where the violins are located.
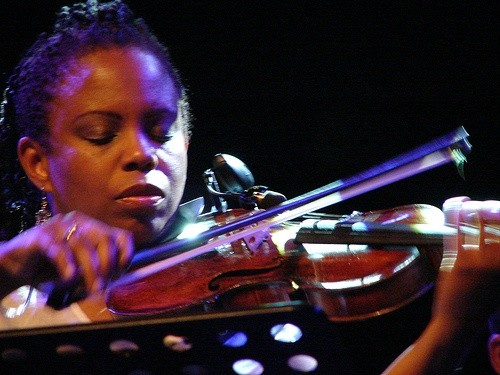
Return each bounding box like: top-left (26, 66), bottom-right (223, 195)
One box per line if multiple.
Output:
top-left (103, 196), bottom-right (499, 323)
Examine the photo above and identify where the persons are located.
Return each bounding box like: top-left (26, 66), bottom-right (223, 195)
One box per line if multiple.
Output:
top-left (0, 0), bottom-right (500, 375)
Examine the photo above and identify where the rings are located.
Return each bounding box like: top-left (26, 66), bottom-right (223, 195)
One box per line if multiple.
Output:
top-left (61, 222), bottom-right (82, 241)
